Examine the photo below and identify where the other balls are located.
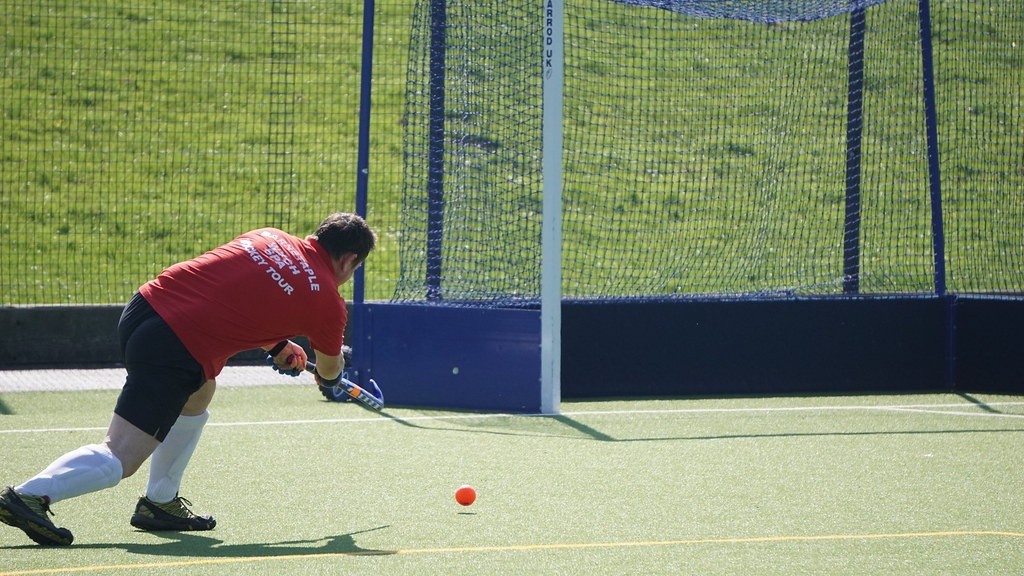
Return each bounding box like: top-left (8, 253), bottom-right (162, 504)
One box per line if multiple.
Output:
top-left (455, 487), bottom-right (475, 506)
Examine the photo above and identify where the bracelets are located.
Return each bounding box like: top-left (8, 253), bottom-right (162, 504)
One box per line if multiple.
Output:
top-left (266, 340), bottom-right (288, 357)
top-left (315, 368), bottom-right (342, 387)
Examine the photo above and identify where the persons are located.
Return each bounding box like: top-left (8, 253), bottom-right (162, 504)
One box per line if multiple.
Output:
top-left (0, 212), bottom-right (376, 547)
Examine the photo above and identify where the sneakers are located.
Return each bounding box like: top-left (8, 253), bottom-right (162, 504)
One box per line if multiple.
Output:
top-left (130, 491), bottom-right (216, 530)
top-left (0, 485), bottom-right (73, 545)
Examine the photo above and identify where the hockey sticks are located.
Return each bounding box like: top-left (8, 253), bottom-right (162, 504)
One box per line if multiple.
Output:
top-left (287, 354), bottom-right (385, 411)
